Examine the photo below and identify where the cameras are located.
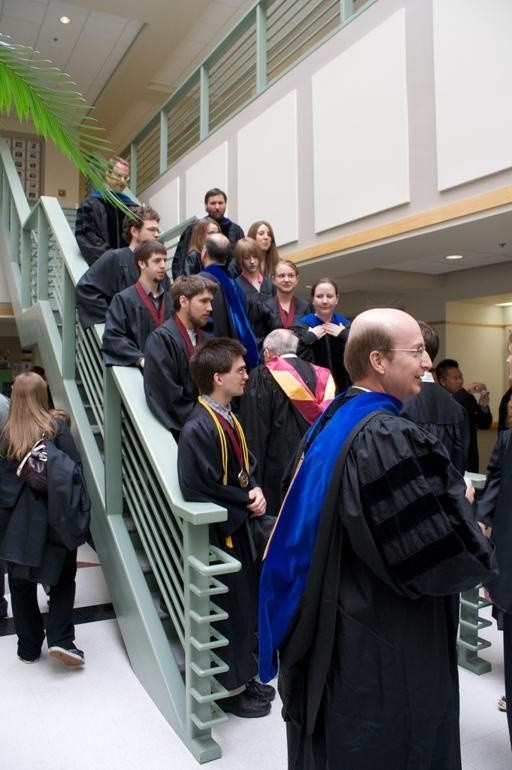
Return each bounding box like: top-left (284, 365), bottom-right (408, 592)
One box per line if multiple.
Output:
top-left (470, 382), bottom-right (483, 393)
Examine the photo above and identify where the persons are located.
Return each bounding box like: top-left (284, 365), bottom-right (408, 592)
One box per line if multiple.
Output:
top-left (1, 365), bottom-right (90, 666)
top-left (74, 155), bottom-right (137, 266)
top-left (76, 189), bottom-right (350, 718)
top-left (477, 429), bottom-right (511, 713)
top-left (402, 320), bottom-right (491, 478)
top-left (259, 308), bottom-right (502, 768)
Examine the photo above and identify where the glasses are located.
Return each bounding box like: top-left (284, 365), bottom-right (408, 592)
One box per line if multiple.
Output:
top-left (382, 345), bottom-right (426, 356)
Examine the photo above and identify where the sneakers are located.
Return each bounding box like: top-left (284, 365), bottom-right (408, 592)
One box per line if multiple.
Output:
top-left (208, 674), bottom-right (276, 719)
top-left (48, 643), bottom-right (86, 666)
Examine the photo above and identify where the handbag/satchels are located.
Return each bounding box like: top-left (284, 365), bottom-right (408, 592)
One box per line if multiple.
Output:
top-left (15, 420), bottom-right (58, 491)
